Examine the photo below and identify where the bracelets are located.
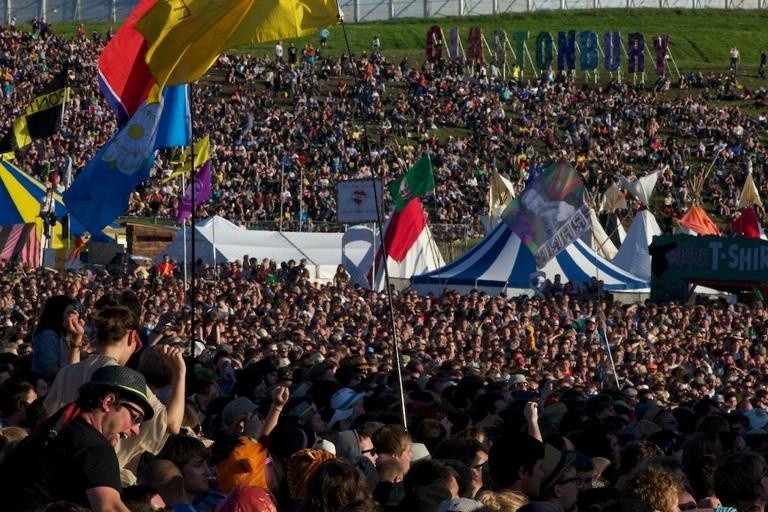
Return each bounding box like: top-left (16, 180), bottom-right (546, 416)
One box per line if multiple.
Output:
top-left (70, 341), bottom-right (83, 349)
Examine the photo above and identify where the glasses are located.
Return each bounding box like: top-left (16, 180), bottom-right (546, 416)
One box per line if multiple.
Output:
top-left (678, 502), bottom-right (699, 511)
top-left (112, 403), bottom-right (145, 429)
top-left (361, 447), bottom-right (376, 456)
top-left (469, 460), bottom-right (488, 469)
top-left (382, 368), bottom-right (392, 373)
top-left (20, 400), bottom-right (32, 409)
top-left (449, 311), bottom-right (454, 312)
top-left (553, 476), bottom-right (581, 486)
top-left (282, 349), bottom-right (289, 354)
top-left (759, 469), bottom-right (768, 480)
top-left (566, 285), bottom-right (571, 287)
top-left (744, 385), bottom-right (751, 389)
top-left (265, 348), bottom-right (279, 353)
top-left (6, 370), bottom-right (15, 377)
top-left (527, 303), bottom-right (532, 306)
top-left (23, 351), bottom-right (32, 356)
top-left (129, 330), bottom-right (143, 353)
top-left (579, 356), bottom-right (587, 358)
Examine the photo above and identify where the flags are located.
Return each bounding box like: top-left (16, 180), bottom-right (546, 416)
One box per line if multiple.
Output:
top-left (97, 0), bottom-right (158, 131)
top-left (134, 0), bottom-right (345, 108)
top-left (163, 135), bottom-right (211, 225)
top-left (1, 69), bottom-right (70, 161)
top-left (386, 151), bottom-right (435, 213)
top-left (63, 83), bottom-right (192, 238)
top-left (500, 155), bottom-right (592, 270)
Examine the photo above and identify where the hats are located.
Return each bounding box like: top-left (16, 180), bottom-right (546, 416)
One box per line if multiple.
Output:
top-left (287, 448), bottom-right (336, 505)
top-left (259, 359), bottom-right (278, 373)
top-left (573, 304), bottom-right (580, 311)
top-left (221, 397), bottom-right (260, 430)
top-left (325, 408), bottom-right (354, 430)
top-left (491, 352), bottom-right (505, 363)
top-left (345, 357), bottom-right (370, 369)
top-left (623, 352), bottom-right (636, 362)
top-left (693, 367), bottom-right (707, 377)
top-left (183, 341), bottom-right (205, 357)
top-left (308, 360), bottom-right (335, 382)
top-left (540, 443), bottom-right (576, 495)
top-left (438, 361), bottom-right (464, 376)
top-left (509, 374), bottom-right (529, 390)
top-left (255, 329), bottom-right (268, 340)
top-left (642, 405), bottom-right (670, 423)
top-left (504, 303), bottom-right (513, 310)
top-left (147, 308), bottom-right (156, 315)
top-left (731, 331), bottom-right (744, 339)
top-left (78, 366), bottom-right (154, 422)
top-left (288, 401), bottom-right (325, 426)
top-left (513, 336), bottom-right (521, 341)
top-left (587, 317), bottom-right (596, 323)
top-left (696, 351), bottom-right (710, 362)
top-left (242, 296), bottom-right (252, 303)
top-left (330, 388), bottom-right (366, 410)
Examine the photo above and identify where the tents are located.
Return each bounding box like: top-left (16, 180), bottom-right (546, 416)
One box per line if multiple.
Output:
top-left (0, 150), bottom-right (128, 271)
top-left (340, 159), bottom-right (767, 310)
top-left (154, 214), bottom-right (376, 287)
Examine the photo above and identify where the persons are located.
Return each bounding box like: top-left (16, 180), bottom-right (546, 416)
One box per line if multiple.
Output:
top-left (11, 139), bottom-right (70, 186)
top-left (120, 486), bottom-right (174, 511)
top-left (593, 156), bottom-right (768, 223)
top-left (1, 381), bottom-right (38, 426)
top-left (164, 434), bottom-right (211, 512)
top-left (277, 307), bottom-right (768, 511)
top-left (210, 306), bottom-right (278, 483)
top-left (51, 253), bottom-right (767, 307)
top-left (42, 307), bottom-right (186, 488)
top-left (386, 46), bottom-right (767, 156)
top-left (422, 156), bottom-right (501, 225)
top-left (0, 426), bottom-right (29, 464)
top-left (136, 460), bottom-right (195, 512)
top-left (70, 83), bottom-right (118, 139)
top-left (1, 258), bottom-right (51, 365)
top-left (39, 188), bottom-right (56, 239)
top-left (181, 366), bottom-right (211, 449)
top-left (192, 41), bottom-right (265, 135)
top-left (32, 296), bottom-right (92, 388)
top-left (212, 135), bottom-right (265, 227)
top-left (23, 373), bottom-right (50, 399)
top-left (0, 366), bottom-right (155, 512)
top-left (122, 148), bottom-right (163, 217)
top-left (0, 82), bottom-right (50, 139)
top-left (71, 138), bottom-right (110, 185)
top-left (321, 27), bottom-right (330, 50)
top-left (0, 15), bottom-right (115, 83)
top-left (144, 306), bottom-right (209, 367)
top-left (265, 36), bottom-right (385, 227)
